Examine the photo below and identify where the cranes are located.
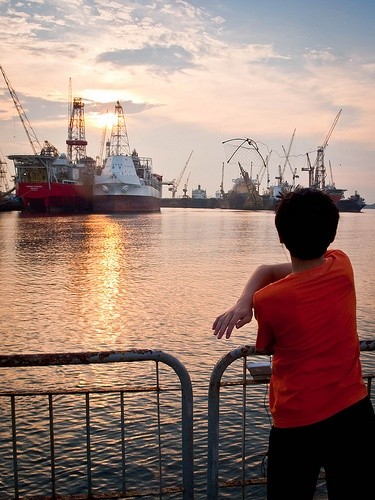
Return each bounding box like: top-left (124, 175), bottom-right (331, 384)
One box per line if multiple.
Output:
top-left (168, 110), bottom-right (355, 197)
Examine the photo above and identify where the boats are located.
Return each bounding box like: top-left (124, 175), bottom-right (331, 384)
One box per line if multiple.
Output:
top-left (258, 184), bottom-right (347, 211)
top-left (93, 153), bottom-right (162, 213)
top-left (161, 184), bottom-right (247, 211)
top-left (0, 191), bottom-right (18, 212)
top-left (6, 141), bottom-right (93, 213)
top-left (339, 191), bottom-right (366, 212)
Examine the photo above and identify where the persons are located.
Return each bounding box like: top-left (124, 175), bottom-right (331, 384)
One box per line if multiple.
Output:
top-left (211, 188), bottom-right (375, 500)
